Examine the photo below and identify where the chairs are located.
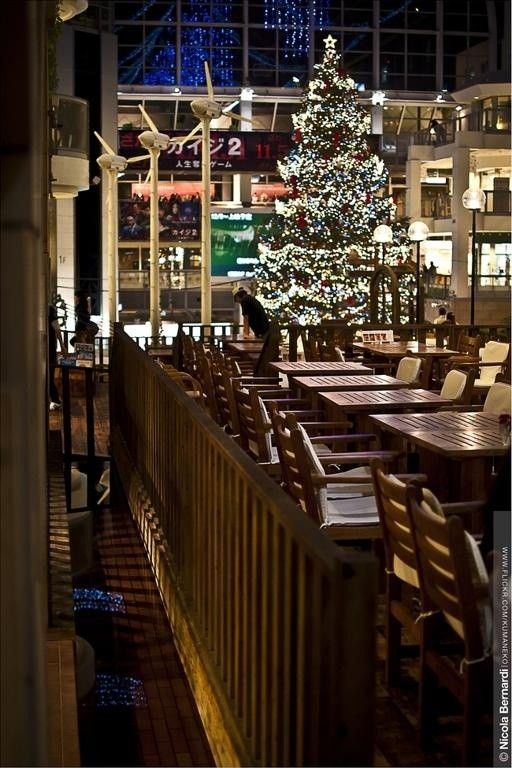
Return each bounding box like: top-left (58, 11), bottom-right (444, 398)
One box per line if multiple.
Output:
top-left (143, 323), bottom-right (512, 767)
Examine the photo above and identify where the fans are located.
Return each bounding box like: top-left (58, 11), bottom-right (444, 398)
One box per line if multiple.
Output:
top-left (92, 60), bottom-right (262, 346)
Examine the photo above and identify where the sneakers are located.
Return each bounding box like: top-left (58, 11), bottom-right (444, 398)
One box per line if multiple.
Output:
top-left (49, 399), bottom-right (63, 411)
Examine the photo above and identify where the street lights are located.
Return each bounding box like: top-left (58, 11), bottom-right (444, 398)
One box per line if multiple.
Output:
top-left (462, 188), bottom-right (486, 325)
top-left (373, 224), bottom-right (392, 322)
top-left (408, 222), bottom-right (429, 339)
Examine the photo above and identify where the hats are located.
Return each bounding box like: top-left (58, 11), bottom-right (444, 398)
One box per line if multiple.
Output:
top-left (232, 286), bottom-right (247, 304)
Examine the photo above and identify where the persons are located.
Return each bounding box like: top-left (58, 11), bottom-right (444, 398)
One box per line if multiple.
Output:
top-left (53, 100), bottom-right (72, 147)
top-left (133, 190), bottom-right (200, 201)
top-left (428, 261), bottom-right (437, 285)
top-left (47, 105), bottom-right (57, 147)
top-left (118, 202), bottom-right (197, 240)
top-left (429, 118), bottom-right (448, 144)
top-left (432, 306), bottom-right (460, 334)
top-left (47, 302), bottom-right (67, 411)
top-left (74, 289), bottom-right (91, 343)
top-left (251, 191), bottom-right (294, 202)
top-left (232, 284), bottom-right (269, 340)
top-left (433, 311), bottom-right (457, 341)
top-left (434, 193), bottom-right (445, 216)
